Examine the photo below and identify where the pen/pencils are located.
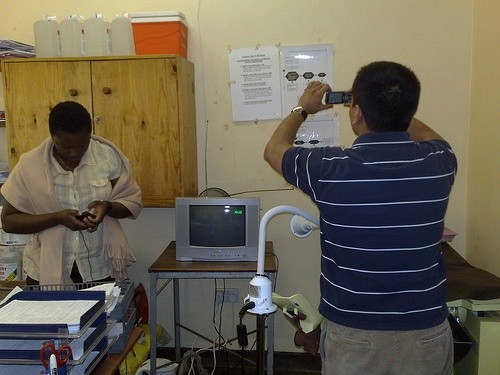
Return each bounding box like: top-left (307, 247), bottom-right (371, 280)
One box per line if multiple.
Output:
top-left (50, 354), bottom-right (58, 375)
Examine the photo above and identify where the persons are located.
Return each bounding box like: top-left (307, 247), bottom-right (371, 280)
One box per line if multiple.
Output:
top-left (264, 61), bottom-right (458, 375)
top-left (0, 100), bottom-right (143, 290)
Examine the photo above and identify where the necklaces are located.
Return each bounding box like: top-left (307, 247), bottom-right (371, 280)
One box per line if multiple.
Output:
top-left (57, 155), bottom-right (72, 170)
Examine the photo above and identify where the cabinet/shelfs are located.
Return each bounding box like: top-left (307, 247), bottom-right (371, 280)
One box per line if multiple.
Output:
top-left (1, 53), bottom-right (198, 209)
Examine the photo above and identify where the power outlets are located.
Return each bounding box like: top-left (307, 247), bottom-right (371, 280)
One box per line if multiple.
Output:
top-left (215, 288), bottom-right (238, 304)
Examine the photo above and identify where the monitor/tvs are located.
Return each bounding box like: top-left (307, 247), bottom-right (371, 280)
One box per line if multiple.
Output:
top-left (174, 196), bottom-right (261, 260)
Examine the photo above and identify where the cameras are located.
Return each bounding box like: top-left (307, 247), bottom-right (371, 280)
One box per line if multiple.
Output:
top-left (324, 90), bottom-right (353, 106)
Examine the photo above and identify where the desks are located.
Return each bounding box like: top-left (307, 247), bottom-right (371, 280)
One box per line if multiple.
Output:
top-left (147, 240), bottom-right (277, 375)
top-left (88, 327), bottom-right (144, 375)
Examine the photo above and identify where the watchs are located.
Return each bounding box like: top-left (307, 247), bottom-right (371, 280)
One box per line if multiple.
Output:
top-left (291, 106), bottom-right (307, 120)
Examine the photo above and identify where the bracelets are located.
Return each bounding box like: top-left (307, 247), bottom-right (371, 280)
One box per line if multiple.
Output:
top-left (103, 201), bottom-right (112, 215)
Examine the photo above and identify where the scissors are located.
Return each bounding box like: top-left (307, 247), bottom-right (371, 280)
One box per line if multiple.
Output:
top-left (40, 342), bottom-right (72, 368)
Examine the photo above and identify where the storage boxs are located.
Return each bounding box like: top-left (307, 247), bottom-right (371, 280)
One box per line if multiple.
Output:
top-left (129, 10), bottom-right (189, 60)
